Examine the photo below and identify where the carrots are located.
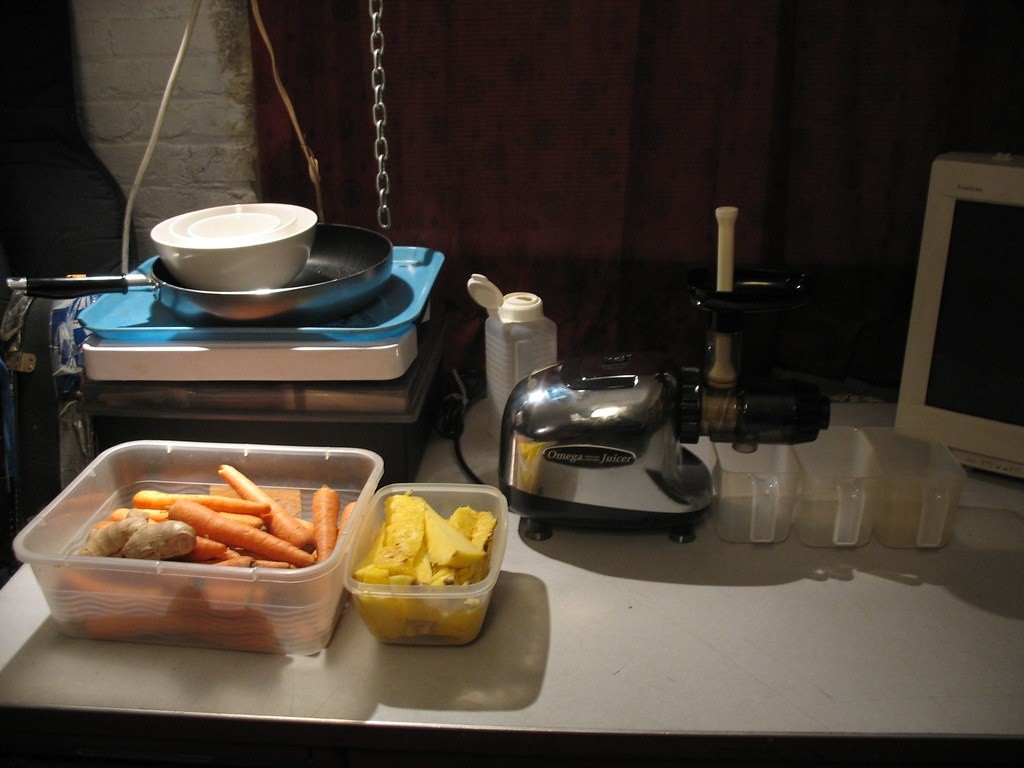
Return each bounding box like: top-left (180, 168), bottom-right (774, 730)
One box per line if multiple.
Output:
top-left (55, 465), bottom-right (353, 653)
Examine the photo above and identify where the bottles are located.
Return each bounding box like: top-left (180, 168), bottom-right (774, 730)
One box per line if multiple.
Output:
top-left (483, 292), bottom-right (558, 444)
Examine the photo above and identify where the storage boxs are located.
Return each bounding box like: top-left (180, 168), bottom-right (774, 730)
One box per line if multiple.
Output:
top-left (710, 425), bottom-right (968, 554)
top-left (12, 438), bottom-right (385, 658)
top-left (338, 482), bottom-right (508, 648)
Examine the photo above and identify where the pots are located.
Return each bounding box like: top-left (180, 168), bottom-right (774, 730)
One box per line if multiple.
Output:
top-left (6, 223), bottom-right (393, 326)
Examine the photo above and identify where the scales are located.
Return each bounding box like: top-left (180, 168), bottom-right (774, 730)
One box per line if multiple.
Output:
top-left (74, 245), bottom-right (456, 424)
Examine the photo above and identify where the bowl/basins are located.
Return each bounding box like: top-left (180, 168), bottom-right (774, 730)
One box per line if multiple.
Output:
top-left (344, 481), bottom-right (509, 646)
top-left (149, 202), bottom-right (319, 292)
top-left (11, 437), bottom-right (385, 657)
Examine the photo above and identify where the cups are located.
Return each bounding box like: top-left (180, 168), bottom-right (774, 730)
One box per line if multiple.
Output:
top-left (791, 426), bottom-right (882, 549)
top-left (713, 441), bottom-right (800, 543)
top-left (860, 423), bottom-right (965, 550)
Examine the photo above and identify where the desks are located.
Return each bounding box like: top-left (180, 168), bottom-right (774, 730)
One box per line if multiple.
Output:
top-left (0, 401), bottom-right (1024, 768)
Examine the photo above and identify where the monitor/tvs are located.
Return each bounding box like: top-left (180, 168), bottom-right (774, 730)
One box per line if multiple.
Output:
top-left (894, 155), bottom-right (1023, 482)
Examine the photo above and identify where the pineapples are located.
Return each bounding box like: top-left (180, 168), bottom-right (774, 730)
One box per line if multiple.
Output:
top-left (350, 493), bottom-right (496, 640)
